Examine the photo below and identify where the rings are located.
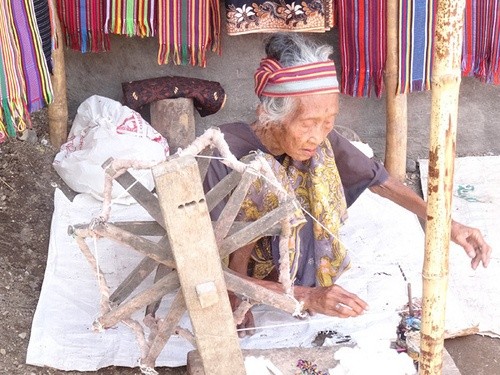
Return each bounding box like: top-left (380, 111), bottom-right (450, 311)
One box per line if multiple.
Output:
top-left (335, 304), bottom-right (341, 311)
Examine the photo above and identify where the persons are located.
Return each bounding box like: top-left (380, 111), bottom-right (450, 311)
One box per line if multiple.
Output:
top-left (202, 30), bottom-right (490, 337)
